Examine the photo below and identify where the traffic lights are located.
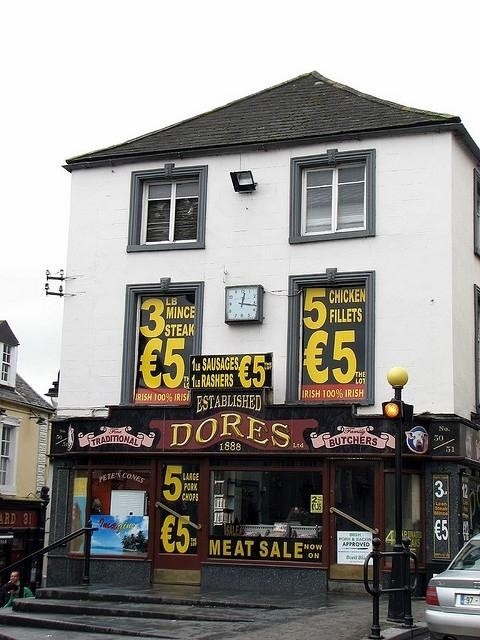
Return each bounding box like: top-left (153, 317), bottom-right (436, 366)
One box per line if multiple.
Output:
top-left (382, 400), bottom-right (403, 424)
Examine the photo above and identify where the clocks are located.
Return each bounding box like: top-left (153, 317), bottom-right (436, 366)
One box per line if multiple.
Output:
top-left (224, 284), bottom-right (263, 324)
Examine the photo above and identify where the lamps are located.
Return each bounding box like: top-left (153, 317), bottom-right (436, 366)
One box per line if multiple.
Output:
top-left (230, 171), bottom-right (256, 193)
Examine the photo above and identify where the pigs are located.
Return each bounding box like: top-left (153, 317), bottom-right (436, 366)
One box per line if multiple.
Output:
top-left (405, 431), bottom-right (430, 452)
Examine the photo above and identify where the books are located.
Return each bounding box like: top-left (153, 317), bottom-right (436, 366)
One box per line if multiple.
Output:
top-left (213, 472), bottom-right (234, 527)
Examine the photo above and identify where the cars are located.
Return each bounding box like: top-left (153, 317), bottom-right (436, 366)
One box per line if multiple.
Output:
top-left (424, 534), bottom-right (480, 640)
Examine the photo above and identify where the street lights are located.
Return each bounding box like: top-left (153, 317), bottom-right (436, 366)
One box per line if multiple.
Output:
top-left (387, 366), bottom-right (416, 622)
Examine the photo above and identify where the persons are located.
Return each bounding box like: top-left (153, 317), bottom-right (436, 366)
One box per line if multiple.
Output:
top-left (0, 570), bottom-right (24, 607)
top-left (69, 502), bottom-right (83, 553)
top-left (2, 581), bottom-right (35, 607)
